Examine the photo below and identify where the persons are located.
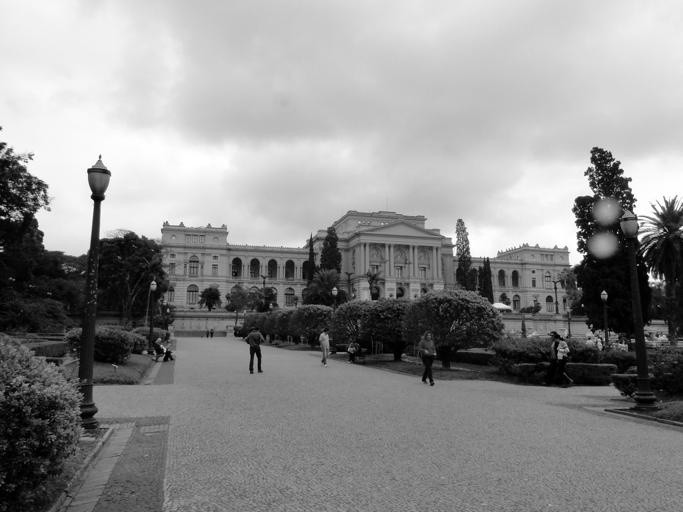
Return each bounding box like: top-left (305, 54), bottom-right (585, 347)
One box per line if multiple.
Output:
top-left (541, 331), bottom-right (574, 386)
top-left (417, 330), bottom-right (437, 385)
top-left (586, 331), bottom-right (669, 352)
top-left (153, 337), bottom-right (173, 361)
top-left (319, 328), bottom-right (330, 368)
top-left (347, 343), bottom-right (362, 364)
top-left (206, 328), bottom-right (215, 339)
top-left (245, 326), bottom-right (265, 374)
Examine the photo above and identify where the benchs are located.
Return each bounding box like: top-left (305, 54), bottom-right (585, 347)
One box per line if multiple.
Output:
top-left (154, 342), bottom-right (177, 362)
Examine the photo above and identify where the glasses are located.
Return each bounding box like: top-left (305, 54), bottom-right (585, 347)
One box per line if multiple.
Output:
top-left (550, 335), bottom-right (553, 336)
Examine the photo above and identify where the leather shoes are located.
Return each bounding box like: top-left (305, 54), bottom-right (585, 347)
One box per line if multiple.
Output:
top-left (250, 370), bottom-right (262, 374)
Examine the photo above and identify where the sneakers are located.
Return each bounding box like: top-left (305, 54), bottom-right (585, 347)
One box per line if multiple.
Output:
top-left (422, 380), bottom-right (435, 386)
top-left (322, 360), bottom-right (329, 367)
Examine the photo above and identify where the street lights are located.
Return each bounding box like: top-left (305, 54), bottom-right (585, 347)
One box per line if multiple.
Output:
top-left (332, 286), bottom-right (338, 312)
top-left (147, 280), bottom-right (157, 356)
top-left (74, 154), bottom-right (112, 430)
top-left (600, 290), bottom-right (612, 351)
top-left (619, 206), bottom-right (661, 413)
